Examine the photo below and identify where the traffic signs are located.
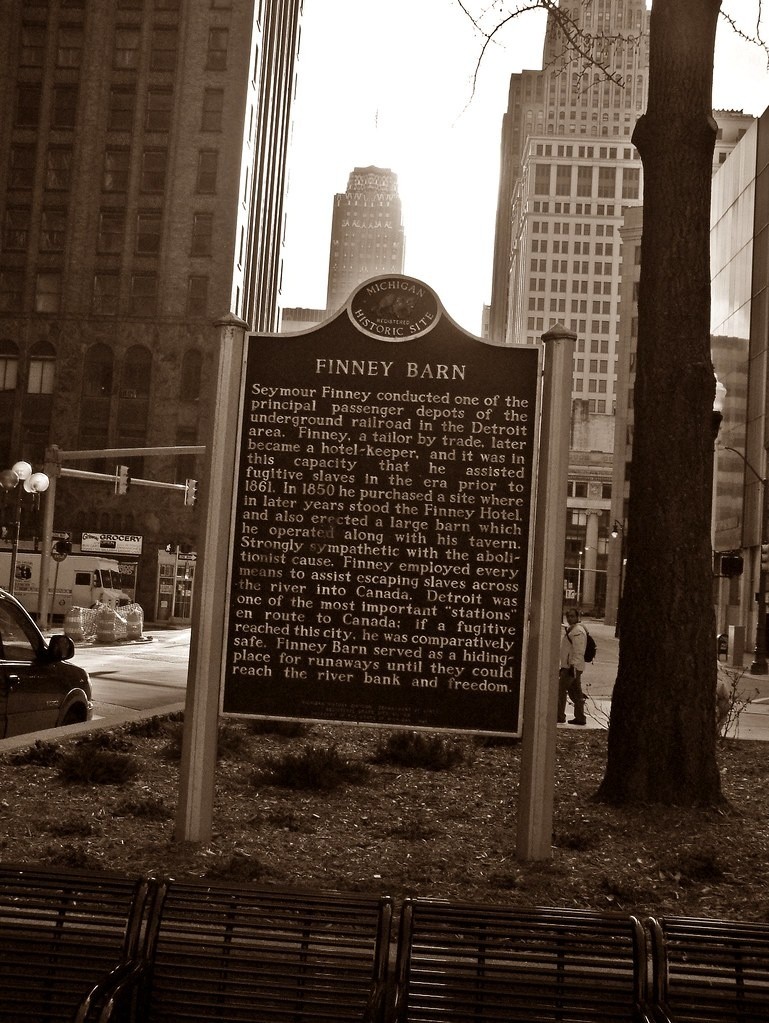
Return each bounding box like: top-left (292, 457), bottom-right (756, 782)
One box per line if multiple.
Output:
top-left (53, 531), bottom-right (72, 542)
top-left (178, 554), bottom-right (197, 561)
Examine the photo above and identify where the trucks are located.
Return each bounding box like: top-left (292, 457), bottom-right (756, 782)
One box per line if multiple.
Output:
top-left (0, 552), bottom-right (133, 617)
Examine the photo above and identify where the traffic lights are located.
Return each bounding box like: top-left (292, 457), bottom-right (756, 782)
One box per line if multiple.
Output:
top-left (165, 542), bottom-right (178, 554)
top-left (187, 479), bottom-right (200, 507)
top-left (762, 544), bottom-right (769, 574)
top-left (0, 523), bottom-right (17, 543)
top-left (55, 543), bottom-right (71, 553)
top-left (719, 555), bottom-right (746, 578)
top-left (119, 466), bottom-right (131, 496)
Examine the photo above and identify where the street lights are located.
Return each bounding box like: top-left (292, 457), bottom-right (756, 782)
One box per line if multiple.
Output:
top-left (711, 377), bottom-right (725, 576)
top-left (0, 461), bottom-right (49, 640)
top-left (612, 519), bottom-right (623, 639)
top-left (585, 546), bottom-right (604, 619)
top-left (577, 551), bottom-right (582, 614)
top-left (716, 445), bottom-right (769, 675)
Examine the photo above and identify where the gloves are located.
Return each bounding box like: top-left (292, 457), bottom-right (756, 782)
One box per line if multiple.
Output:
top-left (568, 665), bottom-right (577, 677)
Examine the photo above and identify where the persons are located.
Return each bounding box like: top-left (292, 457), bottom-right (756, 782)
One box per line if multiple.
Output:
top-left (556, 608), bottom-right (588, 725)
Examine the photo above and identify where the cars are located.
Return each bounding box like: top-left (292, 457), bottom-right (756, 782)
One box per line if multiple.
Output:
top-left (0, 586), bottom-right (94, 734)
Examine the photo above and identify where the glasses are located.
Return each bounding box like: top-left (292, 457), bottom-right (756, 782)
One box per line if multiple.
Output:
top-left (566, 615), bottom-right (577, 619)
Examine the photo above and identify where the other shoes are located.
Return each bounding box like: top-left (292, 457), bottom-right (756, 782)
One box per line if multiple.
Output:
top-left (568, 717), bottom-right (587, 726)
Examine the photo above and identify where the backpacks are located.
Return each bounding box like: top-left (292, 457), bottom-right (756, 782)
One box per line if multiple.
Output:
top-left (566, 625), bottom-right (596, 662)
